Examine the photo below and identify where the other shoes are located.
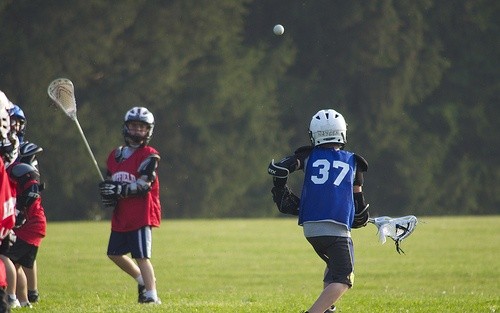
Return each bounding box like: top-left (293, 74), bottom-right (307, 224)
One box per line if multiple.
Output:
top-left (324, 304), bottom-right (336, 313)
top-left (28, 291), bottom-right (40, 303)
top-left (6, 295), bottom-right (21, 309)
top-left (22, 302), bottom-right (33, 309)
top-left (137, 284), bottom-right (157, 304)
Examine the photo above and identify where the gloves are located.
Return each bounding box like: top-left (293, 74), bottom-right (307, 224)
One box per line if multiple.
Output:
top-left (99, 197), bottom-right (118, 208)
top-left (99, 180), bottom-right (129, 200)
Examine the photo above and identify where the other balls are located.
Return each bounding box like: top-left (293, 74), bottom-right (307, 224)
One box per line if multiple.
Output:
top-left (273, 24), bottom-right (285, 35)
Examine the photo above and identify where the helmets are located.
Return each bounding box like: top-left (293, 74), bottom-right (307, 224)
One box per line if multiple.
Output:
top-left (122, 106), bottom-right (155, 146)
top-left (7, 103), bottom-right (26, 142)
top-left (0, 90), bottom-right (16, 138)
top-left (308, 108), bottom-right (347, 147)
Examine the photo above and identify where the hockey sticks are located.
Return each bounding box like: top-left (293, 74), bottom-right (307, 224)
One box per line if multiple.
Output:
top-left (46, 77), bottom-right (107, 184)
top-left (293, 207), bottom-right (416, 242)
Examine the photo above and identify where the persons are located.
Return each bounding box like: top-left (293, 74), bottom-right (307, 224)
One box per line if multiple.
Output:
top-left (0, 88), bottom-right (46, 313)
top-left (268, 109), bottom-right (369, 313)
top-left (98, 106), bottom-right (162, 303)
